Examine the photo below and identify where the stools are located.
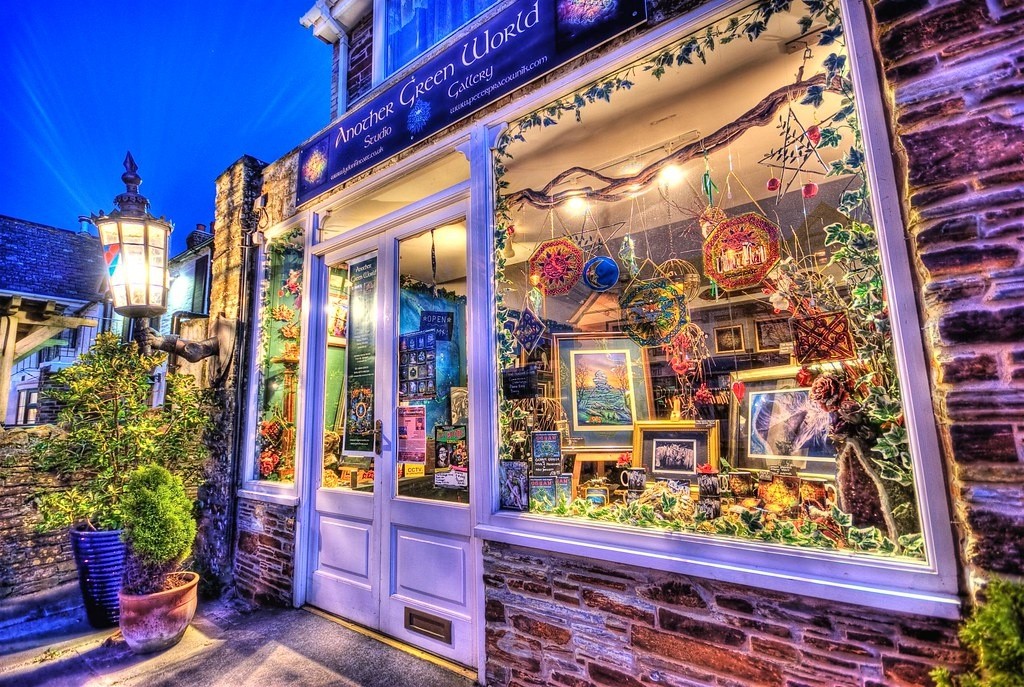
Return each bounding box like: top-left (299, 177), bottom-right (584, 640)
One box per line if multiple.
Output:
top-left (571, 452), bottom-right (633, 488)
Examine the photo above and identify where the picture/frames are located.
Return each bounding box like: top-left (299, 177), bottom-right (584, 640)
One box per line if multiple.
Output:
top-left (755, 316), bottom-right (793, 352)
top-left (333, 377), bottom-right (345, 437)
top-left (631, 419), bottom-right (721, 496)
top-left (327, 274), bottom-right (349, 348)
top-left (727, 358), bottom-right (870, 481)
top-left (713, 324), bottom-right (745, 354)
top-left (520, 331), bottom-right (554, 388)
top-left (551, 331), bottom-right (657, 454)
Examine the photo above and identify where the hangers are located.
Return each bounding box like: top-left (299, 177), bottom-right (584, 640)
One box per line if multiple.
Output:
top-left (654, 388), bottom-right (731, 409)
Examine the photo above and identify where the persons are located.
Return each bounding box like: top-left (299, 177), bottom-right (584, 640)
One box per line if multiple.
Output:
top-left (437, 445), bottom-right (448, 468)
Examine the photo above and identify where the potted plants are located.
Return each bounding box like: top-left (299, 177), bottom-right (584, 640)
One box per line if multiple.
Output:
top-left (29, 328), bottom-right (223, 629)
top-left (117, 464), bottom-right (202, 654)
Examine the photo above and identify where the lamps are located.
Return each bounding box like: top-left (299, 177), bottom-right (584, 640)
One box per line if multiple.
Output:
top-left (501, 238), bottom-right (515, 258)
top-left (92, 149), bottom-right (238, 388)
